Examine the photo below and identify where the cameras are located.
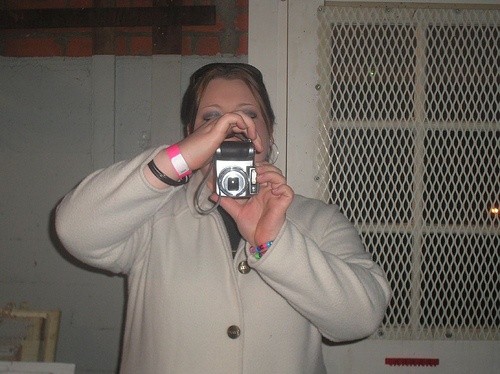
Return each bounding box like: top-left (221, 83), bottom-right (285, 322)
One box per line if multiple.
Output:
top-left (214, 142), bottom-right (257, 198)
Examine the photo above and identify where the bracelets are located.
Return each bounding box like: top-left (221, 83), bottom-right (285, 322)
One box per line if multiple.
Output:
top-left (166, 144), bottom-right (192, 178)
top-left (248, 242), bottom-right (272, 254)
top-left (147, 159), bottom-right (190, 186)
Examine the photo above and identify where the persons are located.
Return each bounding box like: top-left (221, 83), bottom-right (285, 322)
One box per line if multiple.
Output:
top-left (52, 62), bottom-right (392, 374)
